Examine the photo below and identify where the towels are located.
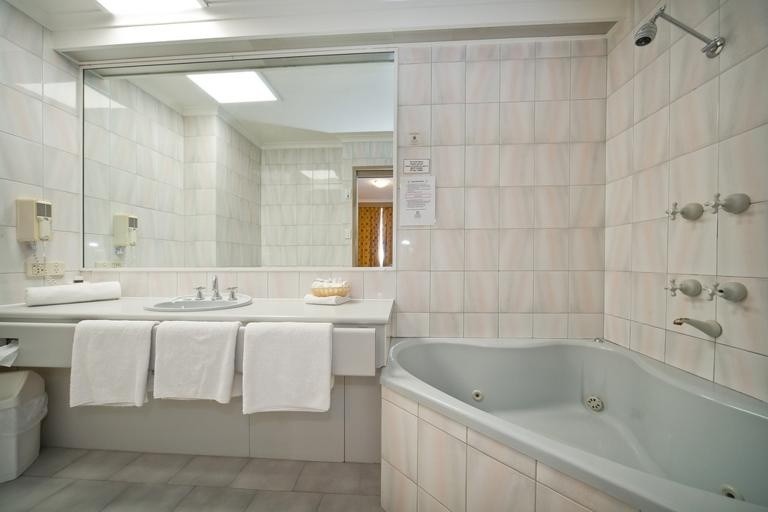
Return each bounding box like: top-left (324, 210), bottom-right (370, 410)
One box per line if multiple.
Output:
top-left (24, 279), bottom-right (122, 307)
top-left (305, 293), bottom-right (352, 307)
top-left (67, 317), bottom-right (336, 415)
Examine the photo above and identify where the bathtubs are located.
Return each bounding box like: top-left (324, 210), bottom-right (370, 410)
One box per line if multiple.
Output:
top-left (380, 338), bottom-right (768, 512)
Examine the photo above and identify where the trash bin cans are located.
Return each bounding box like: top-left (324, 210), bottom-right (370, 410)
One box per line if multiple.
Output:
top-left (0, 371), bottom-right (46, 483)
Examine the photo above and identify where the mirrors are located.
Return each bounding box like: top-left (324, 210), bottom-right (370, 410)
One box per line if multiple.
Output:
top-left (75, 43), bottom-right (400, 272)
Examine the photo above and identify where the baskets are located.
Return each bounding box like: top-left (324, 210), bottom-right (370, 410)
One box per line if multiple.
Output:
top-left (312, 287), bottom-right (348, 296)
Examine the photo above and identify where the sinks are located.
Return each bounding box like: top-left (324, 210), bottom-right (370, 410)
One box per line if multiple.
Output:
top-left (144, 295), bottom-right (252, 312)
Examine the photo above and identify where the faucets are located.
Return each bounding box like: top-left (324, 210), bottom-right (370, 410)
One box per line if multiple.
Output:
top-left (673, 317), bottom-right (723, 339)
top-left (211, 275), bottom-right (223, 300)
